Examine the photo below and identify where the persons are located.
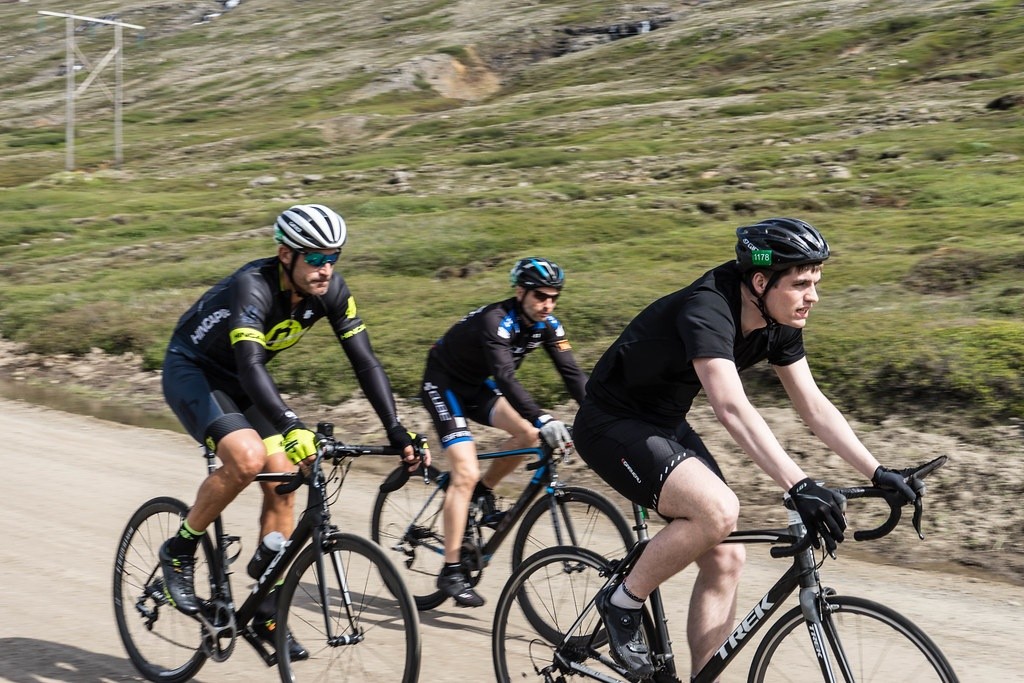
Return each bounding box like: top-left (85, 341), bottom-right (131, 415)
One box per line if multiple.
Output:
top-left (157, 204), bottom-right (433, 664)
top-left (422, 256), bottom-right (593, 608)
top-left (573, 216), bottom-right (924, 682)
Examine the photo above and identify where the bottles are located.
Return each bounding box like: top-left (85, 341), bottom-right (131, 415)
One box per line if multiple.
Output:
top-left (247, 530), bottom-right (286, 579)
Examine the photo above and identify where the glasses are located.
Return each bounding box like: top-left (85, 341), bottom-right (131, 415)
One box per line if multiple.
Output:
top-left (296, 248), bottom-right (341, 267)
top-left (534, 290), bottom-right (559, 303)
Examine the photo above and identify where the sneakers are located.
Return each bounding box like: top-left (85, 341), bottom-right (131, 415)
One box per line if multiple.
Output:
top-left (159, 537), bottom-right (201, 615)
top-left (472, 495), bottom-right (496, 515)
top-left (437, 568), bottom-right (485, 607)
top-left (595, 584), bottom-right (655, 680)
top-left (251, 613), bottom-right (309, 660)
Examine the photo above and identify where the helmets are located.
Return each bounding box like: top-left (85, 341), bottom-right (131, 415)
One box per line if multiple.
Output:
top-left (273, 204), bottom-right (347, 255)
top-left (735, 217), bottom-right (830, 271)
top-left (510, 257), bottom-right (565, 287)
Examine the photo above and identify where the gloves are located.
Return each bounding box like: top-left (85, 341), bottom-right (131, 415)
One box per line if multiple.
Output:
top-left (540, 419), bottom-right (571, 452)
top-left (788, 477), bottom-right (848, 550)
top-left (871, 465), bottom-right (924, 507)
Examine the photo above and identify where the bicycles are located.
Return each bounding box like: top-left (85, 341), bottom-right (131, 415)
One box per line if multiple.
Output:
top-left (491, 454), bottom-right (960, 683)
top-left (113, 419), bottom-right (430, 683)
top-left (371, 430), bottom-right (636, 649)
